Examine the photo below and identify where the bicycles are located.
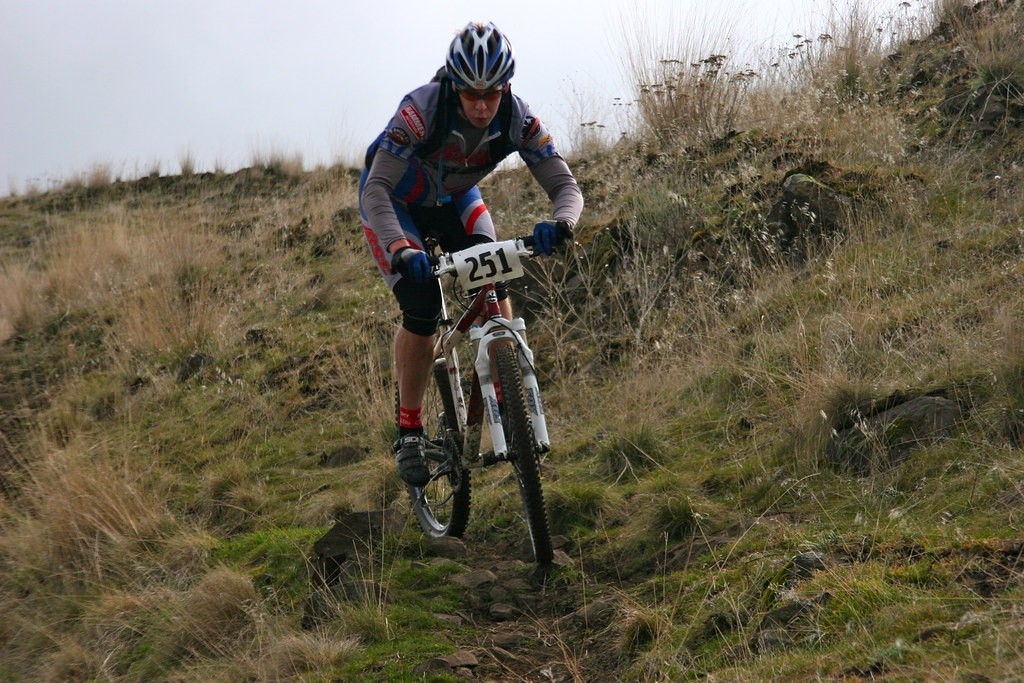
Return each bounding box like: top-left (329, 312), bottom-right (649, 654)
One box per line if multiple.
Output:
top-left (394, 221), bottom-right (577, 568)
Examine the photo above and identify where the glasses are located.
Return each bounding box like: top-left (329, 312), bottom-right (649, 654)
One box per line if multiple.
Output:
top-left (453, 81), bottom-right (508, 100)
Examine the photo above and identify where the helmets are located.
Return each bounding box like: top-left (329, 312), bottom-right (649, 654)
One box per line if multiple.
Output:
top-left (444, 21), bottom-right (514, 90)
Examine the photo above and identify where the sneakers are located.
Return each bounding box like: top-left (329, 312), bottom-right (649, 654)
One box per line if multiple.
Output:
top-left (392, 433), bottom-right (431, 487)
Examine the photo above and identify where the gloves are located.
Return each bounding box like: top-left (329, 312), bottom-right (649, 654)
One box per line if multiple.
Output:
top-left (389, 247), bottom-right (433, 284)
top-left (531, 219), bottom-right (571, 256)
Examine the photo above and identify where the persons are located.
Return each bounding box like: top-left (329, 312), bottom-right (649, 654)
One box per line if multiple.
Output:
top-left (357, 21), bottom-right (584, 487)
